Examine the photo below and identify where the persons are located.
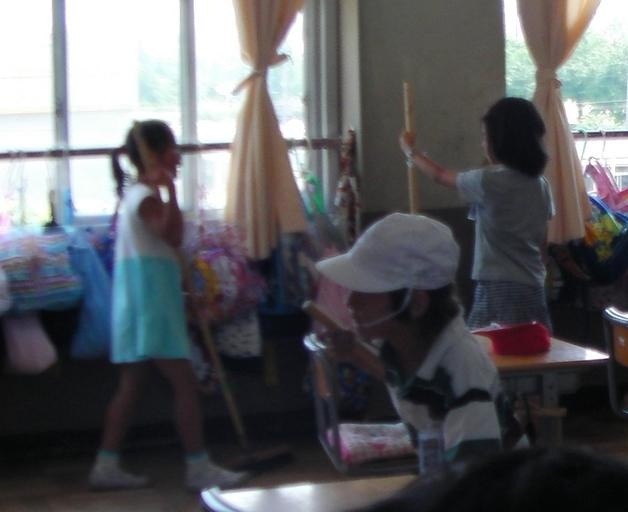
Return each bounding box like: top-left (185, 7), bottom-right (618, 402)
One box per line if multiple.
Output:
top-left (311, 209), bottom-right (529, 466)
top-left (398, 95), bottom-right (555, 451)
top-left (85, 119), bottom-right (248, 489)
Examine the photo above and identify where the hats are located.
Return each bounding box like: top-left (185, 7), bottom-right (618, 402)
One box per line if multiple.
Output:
top-left (316, 213), bottom-right (460, 294)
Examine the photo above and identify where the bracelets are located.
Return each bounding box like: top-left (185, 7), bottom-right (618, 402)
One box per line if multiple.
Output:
top-left (405, 147), bottom-right (420, 168)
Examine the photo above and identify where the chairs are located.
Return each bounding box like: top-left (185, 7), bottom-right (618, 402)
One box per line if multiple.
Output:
top-left (600, 305), bottom-right (628, 422)
top-left (304, 334), bottom-right (420, 475)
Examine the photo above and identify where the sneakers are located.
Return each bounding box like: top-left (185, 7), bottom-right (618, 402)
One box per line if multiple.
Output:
top-left (89, 465), bottom-right (146, 488)
top-left (187, 464), bottom-right (251, 491)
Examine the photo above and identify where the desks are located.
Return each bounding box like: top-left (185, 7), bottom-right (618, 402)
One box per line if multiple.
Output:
top-left (469, 328), bottom-right (612, 449)
